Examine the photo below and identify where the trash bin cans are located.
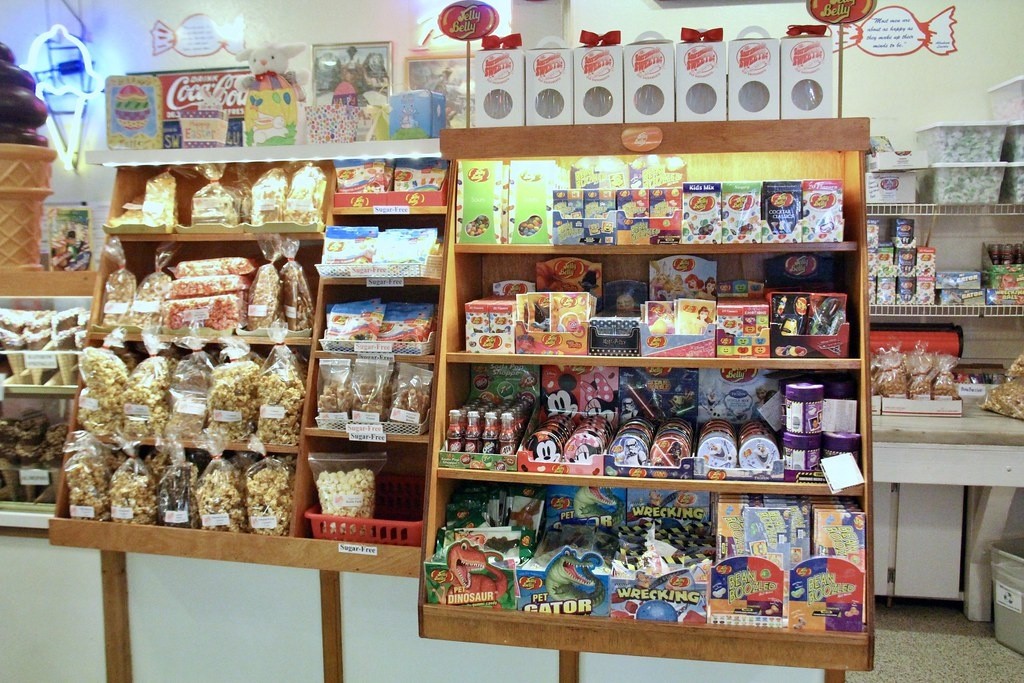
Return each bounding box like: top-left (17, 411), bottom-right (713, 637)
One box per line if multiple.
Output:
top-left (989, 532), bottom-right (1024, 655)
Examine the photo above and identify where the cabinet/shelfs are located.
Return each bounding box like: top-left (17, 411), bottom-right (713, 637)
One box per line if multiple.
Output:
top-left (865, 204), bottom-right (1024, 621)
top-left (418, 117), bottom-right (877, 673)
top-left (0, 294), bottom-right (93, 538)
top-left (49, 138), bottom-right (455, 579)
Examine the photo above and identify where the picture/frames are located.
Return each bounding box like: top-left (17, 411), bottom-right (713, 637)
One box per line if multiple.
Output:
top-left (406, 55), bottom-right (476, 128)
top-left (311, 42), bottom-right (392, 107)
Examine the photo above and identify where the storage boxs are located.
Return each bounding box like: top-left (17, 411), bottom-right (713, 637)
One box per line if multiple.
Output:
top-left (471, 24), bottom-right (835, 129)
top-left (314, 255), bottom-right (443, 277)
top-left (304, 90), bottom-right (446, 144)
top-left (457, 159), bottom-right (847, 247)
top-left (318, 330), bottom-right (435, 355)
top-left (424, 480), bottom-right (865, 633)
top-left (989, 538), bottom-right (1024, 655)
top-left (436, 364), bottom-right (866, 493)
top-left (180, 110), bottom-right (228, 148)
top-left (304, 474), bottom-right (424, 547)
top-left (335, 164), bottom-right (450, 207)
top-left (316, 408), bottom-right (429, 434)
top-left (868, 217), bottom-right (1024, 307)
top-left (466, 252), bottom-right (849, 357)
top-left (865, 75), bottom-right (1024, 206)
